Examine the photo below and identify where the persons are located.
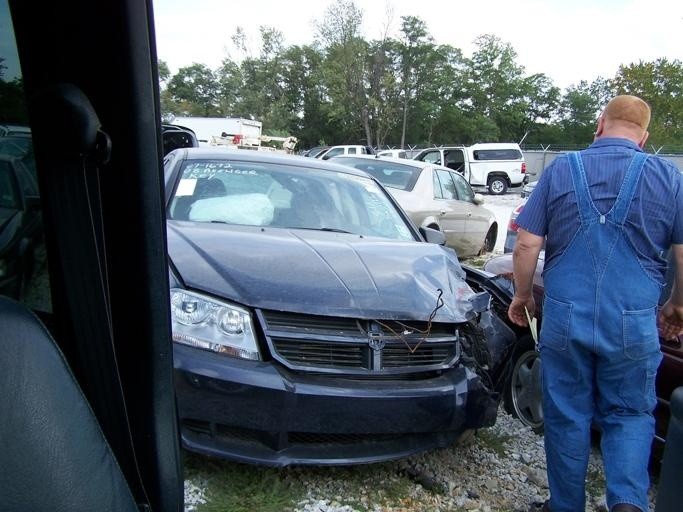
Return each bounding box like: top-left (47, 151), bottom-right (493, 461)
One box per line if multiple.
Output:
top-left (507, 94), bottom-right (682, 512)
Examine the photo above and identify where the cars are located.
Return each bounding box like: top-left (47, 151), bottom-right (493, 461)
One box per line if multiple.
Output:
top-left (519, 178), bottom-right (540, 199)
top-left (483, 242), bottom-right (679, 468)
top-left (2, 128), bottom-right (46, 305)
top-left (502, 199), bottom-right (548, 255)
top-left (160, 145), bottom-right (516, 466)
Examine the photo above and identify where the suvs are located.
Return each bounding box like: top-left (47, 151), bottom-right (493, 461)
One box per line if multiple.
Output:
top-left (467, 142), bottom-right (527, 195)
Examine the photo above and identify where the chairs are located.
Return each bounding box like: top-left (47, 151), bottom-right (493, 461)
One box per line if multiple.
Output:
top-left (173, 177), bottom-right (228, 221)
top-left (1, 293), bottom-right (142, 512)
